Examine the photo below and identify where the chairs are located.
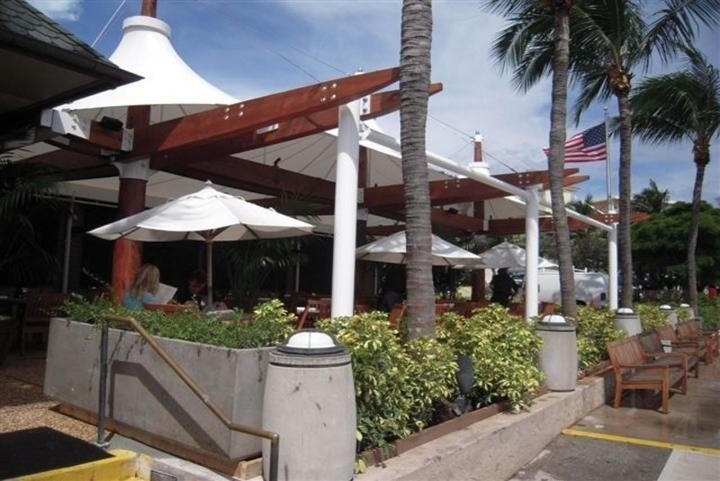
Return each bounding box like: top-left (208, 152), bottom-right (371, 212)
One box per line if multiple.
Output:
top-left (142, 300), bottom-right (195, 315)
top-left (605, 316), bottom-right (719, 414)
top-left (84, 286), bottom-right (112, 306)
top-left (1, 286), bottom-right (68, 355)
top-left (197, 286), bottom-right (370, 329)
top-left (385, 299), bottom-right (595, 330)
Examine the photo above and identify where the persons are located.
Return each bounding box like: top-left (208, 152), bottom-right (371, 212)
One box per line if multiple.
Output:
top-left (123, 264), bottom-right (163, 310)
top-left (487, 268), bottom-right (519, 306)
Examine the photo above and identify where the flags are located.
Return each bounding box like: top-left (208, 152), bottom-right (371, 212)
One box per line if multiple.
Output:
top-left (542, 122), bottom-right (606, 164)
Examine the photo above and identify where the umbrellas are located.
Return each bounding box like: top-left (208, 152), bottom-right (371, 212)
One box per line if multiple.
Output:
top-left (355, 227), bottom-right (486, 304)
top-left (460, 241), bottom-right (556, 272)
top-left (85, 181), bottom-right (318, 313)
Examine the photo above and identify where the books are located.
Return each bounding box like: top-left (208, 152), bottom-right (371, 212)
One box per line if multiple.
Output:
top-left (156, 279), bottom-right (182, 304)
